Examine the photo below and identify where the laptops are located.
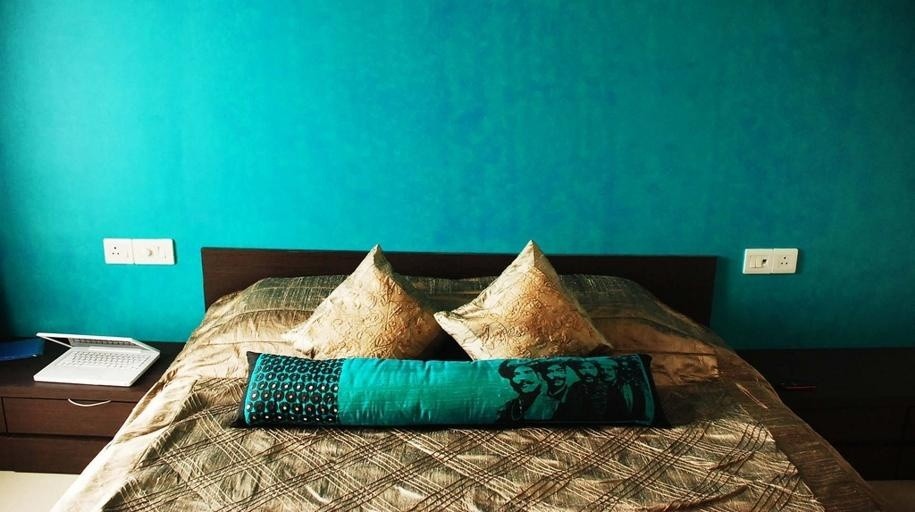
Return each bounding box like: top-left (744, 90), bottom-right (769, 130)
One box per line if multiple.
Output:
top-left (33, 332), bottom-right (161, 387)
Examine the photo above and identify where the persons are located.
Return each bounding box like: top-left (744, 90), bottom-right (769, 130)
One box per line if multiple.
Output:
top-left (495, 357), bottom-right (645, 425)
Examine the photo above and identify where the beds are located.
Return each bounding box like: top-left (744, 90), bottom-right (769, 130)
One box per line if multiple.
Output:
top-left (49, 243), bottom-right (901, 512)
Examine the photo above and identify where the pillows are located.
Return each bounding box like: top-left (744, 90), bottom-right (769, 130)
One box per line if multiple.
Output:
top-left (281, 245), bottom-right (444, 359)
top-left (432, 238), bottom-right (616, 357)
top-left (228, 350), bottom-right (672, 431)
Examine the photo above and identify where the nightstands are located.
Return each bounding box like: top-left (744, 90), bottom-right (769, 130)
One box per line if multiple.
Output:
top-left (732, 346), bottom-right (914, 479)
top-left (0, 334), bottom-right (188, 473)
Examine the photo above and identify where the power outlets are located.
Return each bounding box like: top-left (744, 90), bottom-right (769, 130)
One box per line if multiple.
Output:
top-left (772, 248), bottom-right (800, 274)
top-left (102, 237), bottom-right (134, 266)
